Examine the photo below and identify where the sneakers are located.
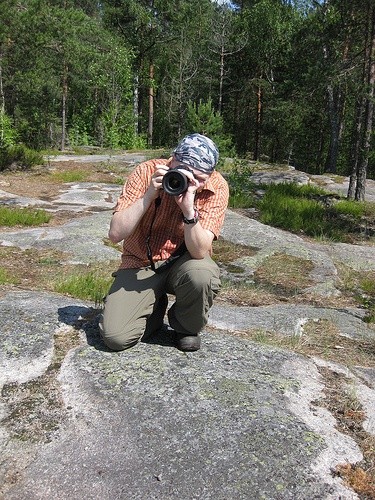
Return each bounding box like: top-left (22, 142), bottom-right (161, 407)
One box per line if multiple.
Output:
top-left (156, 293), bottom-right (168, 327)
top-left (176, 333), bottom-right (200, 351)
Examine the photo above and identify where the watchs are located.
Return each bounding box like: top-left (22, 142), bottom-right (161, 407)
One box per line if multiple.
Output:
top-left (182, 209), bottom-right (198, 225)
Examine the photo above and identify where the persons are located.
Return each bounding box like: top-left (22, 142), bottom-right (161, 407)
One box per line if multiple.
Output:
top-left (98, 132), bottom-right (229, 352)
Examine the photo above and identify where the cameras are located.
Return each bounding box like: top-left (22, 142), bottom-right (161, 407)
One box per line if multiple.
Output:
top-left (162, 165), bottom-right (193, 196)
